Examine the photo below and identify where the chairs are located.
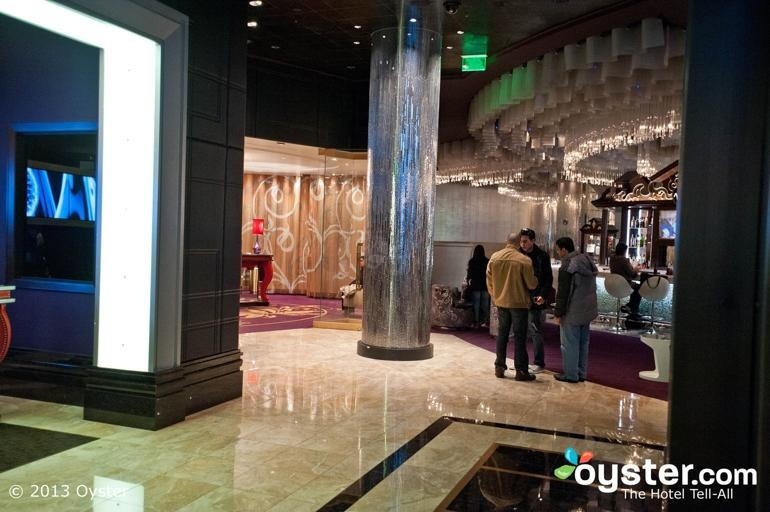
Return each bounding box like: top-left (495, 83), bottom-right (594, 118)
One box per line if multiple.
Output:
top-left (603, 273), bottom-right (670, 341)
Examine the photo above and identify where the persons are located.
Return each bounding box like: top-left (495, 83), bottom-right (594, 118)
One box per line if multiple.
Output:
top-left (552, 236), bottom-right (598, 384)
top-left (465, 244), bottom-right (491, 329)
top-left (485, 231), bottom-right (540, 382)
top-left (509, 228), bottom-right (553, 374)
top-left (341, 255), bottom-right (364, 316)
top-left (609, 241), bottom-right (642, 317)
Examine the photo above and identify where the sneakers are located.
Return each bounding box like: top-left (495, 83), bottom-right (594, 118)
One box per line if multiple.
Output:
top-left (495, 364), bottom-right (584, 383)
top-left (621, 305), bottom-right (643, 317)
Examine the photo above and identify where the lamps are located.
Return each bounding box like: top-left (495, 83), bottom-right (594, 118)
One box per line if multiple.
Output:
top-left (251, 218), bottom-right (265, 254)
